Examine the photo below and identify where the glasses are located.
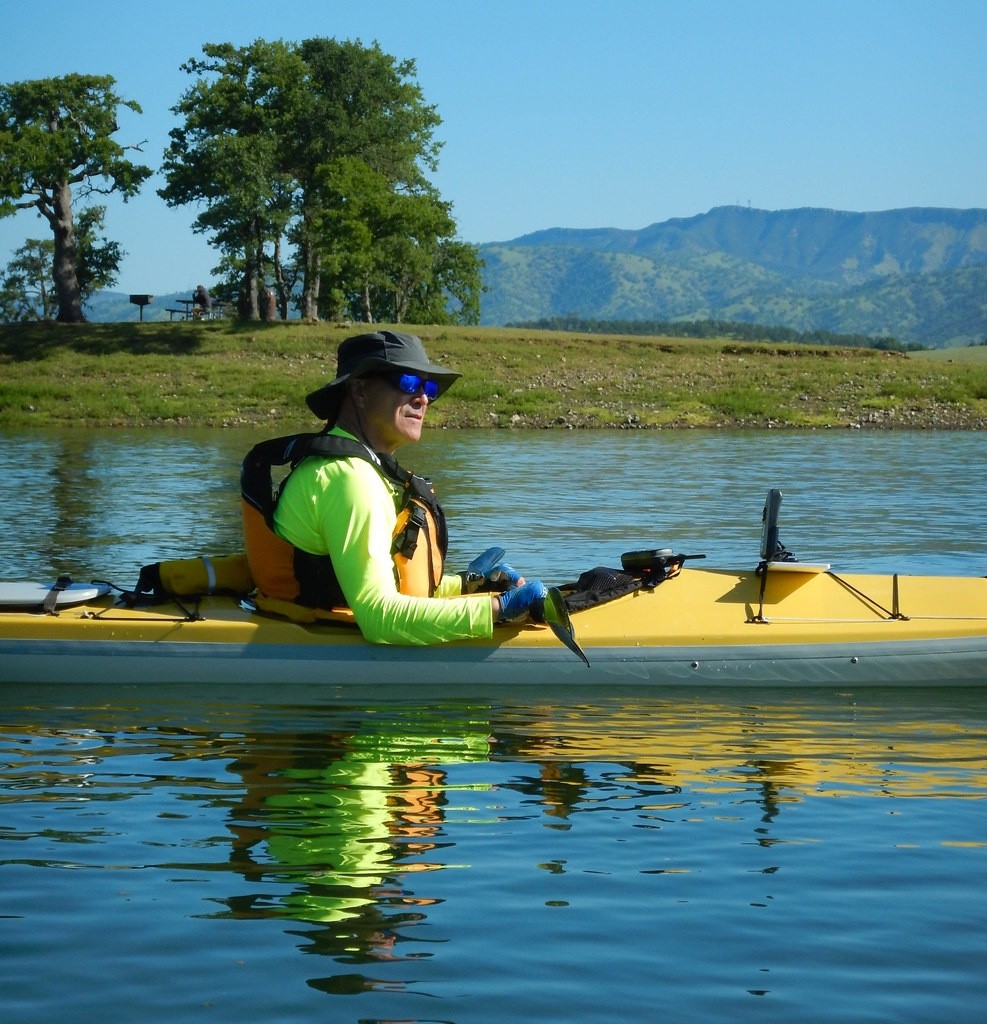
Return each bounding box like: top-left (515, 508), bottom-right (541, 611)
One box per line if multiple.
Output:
top-left (372, 365), bottom-right (439, 400)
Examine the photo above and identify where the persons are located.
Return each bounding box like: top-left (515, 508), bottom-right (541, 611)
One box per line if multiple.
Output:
top-left (191, 285), bottom-right (212, 320)
top-left (241, 331), bottom-right (549, 647)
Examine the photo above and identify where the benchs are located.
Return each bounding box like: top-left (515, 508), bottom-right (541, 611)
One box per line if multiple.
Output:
top-left (165, 309), bottom-right (223, 321)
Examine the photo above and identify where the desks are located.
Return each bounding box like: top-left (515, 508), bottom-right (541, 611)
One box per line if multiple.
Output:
top-left (175, 300), bottom-right (227, 322)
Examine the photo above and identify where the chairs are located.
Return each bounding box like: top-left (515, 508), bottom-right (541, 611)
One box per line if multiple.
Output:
top-left (125, 552), bottom-right (262, 615)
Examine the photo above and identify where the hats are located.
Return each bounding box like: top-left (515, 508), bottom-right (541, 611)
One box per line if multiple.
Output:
top-left (306, 330), bottom-right (464, 420)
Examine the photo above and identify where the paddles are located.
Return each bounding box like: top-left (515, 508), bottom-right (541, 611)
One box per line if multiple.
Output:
top-left (462, 547), bottom-right (593, 668)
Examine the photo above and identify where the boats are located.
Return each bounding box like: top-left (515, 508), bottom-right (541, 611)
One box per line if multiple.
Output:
top-left (2, 488), bottom-right (987, 695)
